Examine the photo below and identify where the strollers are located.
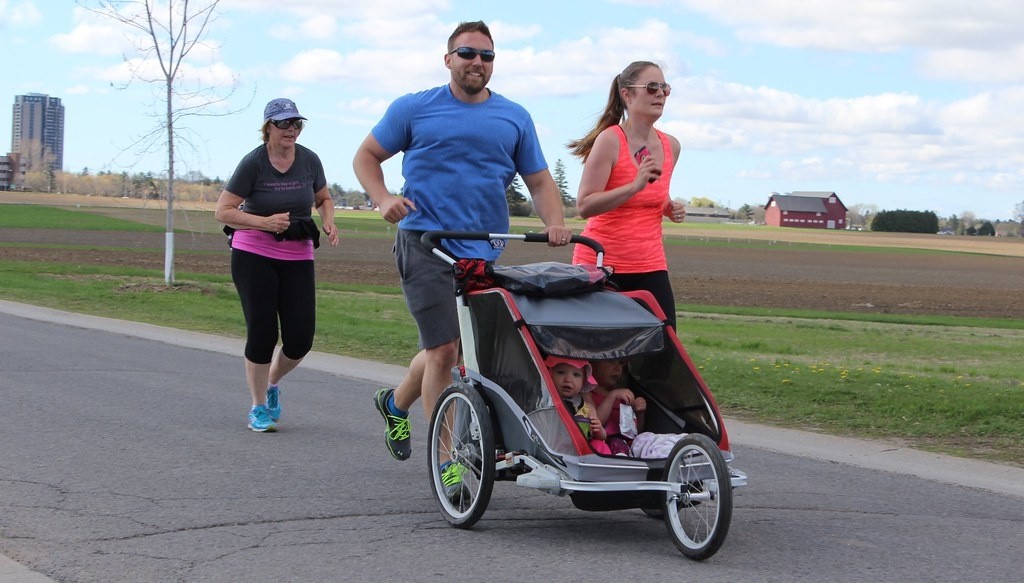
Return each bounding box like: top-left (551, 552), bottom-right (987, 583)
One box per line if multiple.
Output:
top-left (420, 230), bottom-right (748, 562)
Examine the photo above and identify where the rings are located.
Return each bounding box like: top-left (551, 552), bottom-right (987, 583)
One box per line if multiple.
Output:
top-left (561, 238), bottom-right (567, 242)
top-left (677, 215), bottom-right (680, 219)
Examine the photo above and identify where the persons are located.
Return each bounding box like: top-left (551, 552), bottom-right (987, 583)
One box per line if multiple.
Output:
top-left (571, 59), bottom-right (750, 338)
top-left (211, 97), bottom-right (343, 434)
top-left (348, 19), bottom-right (576, 506)
top-left (543, 355), bottom-right (656, 461)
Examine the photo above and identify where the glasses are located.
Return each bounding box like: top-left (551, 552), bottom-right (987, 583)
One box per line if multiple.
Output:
top-left (268, 120), bottom-right (302, 130)
top-left (449, 47), bottom-right (495, 63)
top-left (626, 82), bottom-right (671, 97)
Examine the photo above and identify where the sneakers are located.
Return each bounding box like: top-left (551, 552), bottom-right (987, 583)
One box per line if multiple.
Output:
top-left (374, 389), bottom-right (412, 461)
top-left (266, 386), bottom-right (281, 422)
top-left (247, 404), bottom-right (278, 432)
top-left (440, 460), bottom-right (471, 504)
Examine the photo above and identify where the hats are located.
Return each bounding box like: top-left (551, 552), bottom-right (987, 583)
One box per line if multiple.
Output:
top-left (264, 98), bottom-right (308, 120)
top-left (544, 341), bottom-right (598, 393)
